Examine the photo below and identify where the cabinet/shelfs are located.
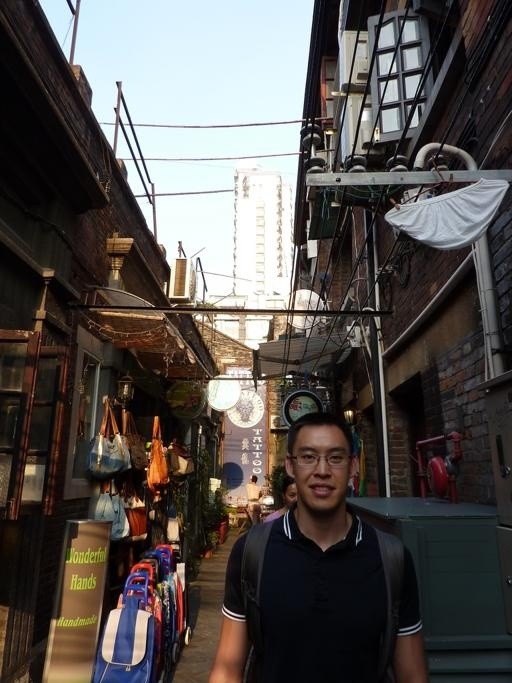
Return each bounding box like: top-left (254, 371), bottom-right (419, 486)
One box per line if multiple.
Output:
top-left (346, 495), bottom-right (511, 683)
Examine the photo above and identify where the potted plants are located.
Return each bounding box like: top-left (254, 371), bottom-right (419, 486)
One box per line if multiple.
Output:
top-left (194, 484), bottom-right (230, 559)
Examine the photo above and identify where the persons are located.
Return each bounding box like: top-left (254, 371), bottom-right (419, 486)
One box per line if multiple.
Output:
top-left (261, 477), bottom-right (297, 525)
top-left (245, 475), bottom-right (274, 510)
top-left (207, 412), bottom-right (428, 683)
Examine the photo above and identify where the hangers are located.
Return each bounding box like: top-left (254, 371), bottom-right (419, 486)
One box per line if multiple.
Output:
top-left (398, 153), bottom-right (478, 206)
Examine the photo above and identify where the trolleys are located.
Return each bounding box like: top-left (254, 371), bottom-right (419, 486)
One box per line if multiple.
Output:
top-left (82, 540), bottom-right (192, 683)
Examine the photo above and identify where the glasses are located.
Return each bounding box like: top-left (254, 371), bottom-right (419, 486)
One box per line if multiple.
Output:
top-left (290, 453), bottom-right (352, 466)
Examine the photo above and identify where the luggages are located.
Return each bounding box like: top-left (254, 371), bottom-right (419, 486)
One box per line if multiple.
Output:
top-left (91, 544), bottom-right (192, 683)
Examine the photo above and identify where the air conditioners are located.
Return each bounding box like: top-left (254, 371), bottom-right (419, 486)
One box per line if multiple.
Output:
top-left (166, 258), bottom-right (197, 303)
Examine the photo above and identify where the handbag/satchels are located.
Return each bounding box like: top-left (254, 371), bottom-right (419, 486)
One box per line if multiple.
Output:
top-left (85, 433), bottom-right (195, 542)
